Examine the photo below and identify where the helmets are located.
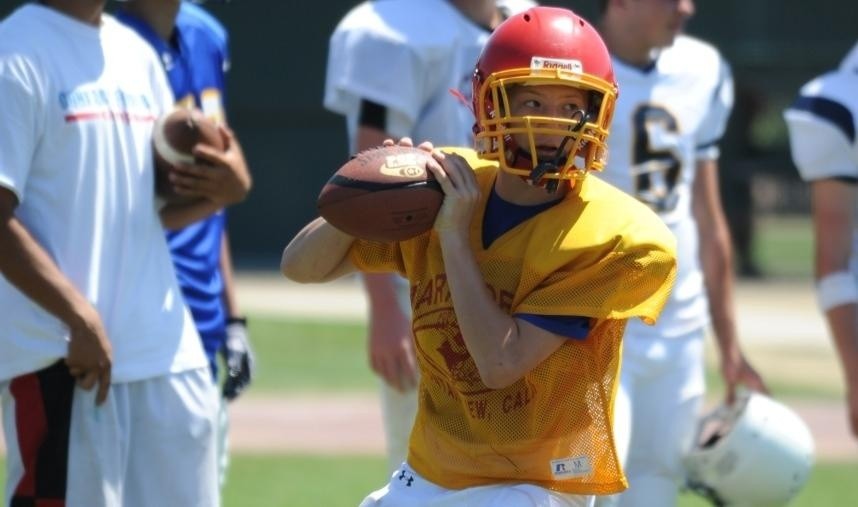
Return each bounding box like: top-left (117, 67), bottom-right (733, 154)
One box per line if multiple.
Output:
top-left (470, 4), bottom-right (620, 163)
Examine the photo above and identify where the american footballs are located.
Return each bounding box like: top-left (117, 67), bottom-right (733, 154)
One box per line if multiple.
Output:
top-left (312, 145), bottom-right (469, 238)
top-left (144, 100), bottom-right (234, 205)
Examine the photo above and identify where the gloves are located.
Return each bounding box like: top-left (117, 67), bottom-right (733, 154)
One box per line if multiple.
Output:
top-left (218, 321), bottom-right (256, 400)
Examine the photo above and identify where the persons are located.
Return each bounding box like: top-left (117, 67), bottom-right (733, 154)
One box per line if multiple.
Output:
top-left (325, 4), bottom-right (542, 478)
top-left (782, 39), bottom-right (858, 438)
top-left (93, 0), bottom-right (256, 488)
top-left (282, 6), bottom-right (678, 507)
top-left (557, 0), bottom-right (770, 506)
top-left (0, 0), bottom-right (253, 507)
